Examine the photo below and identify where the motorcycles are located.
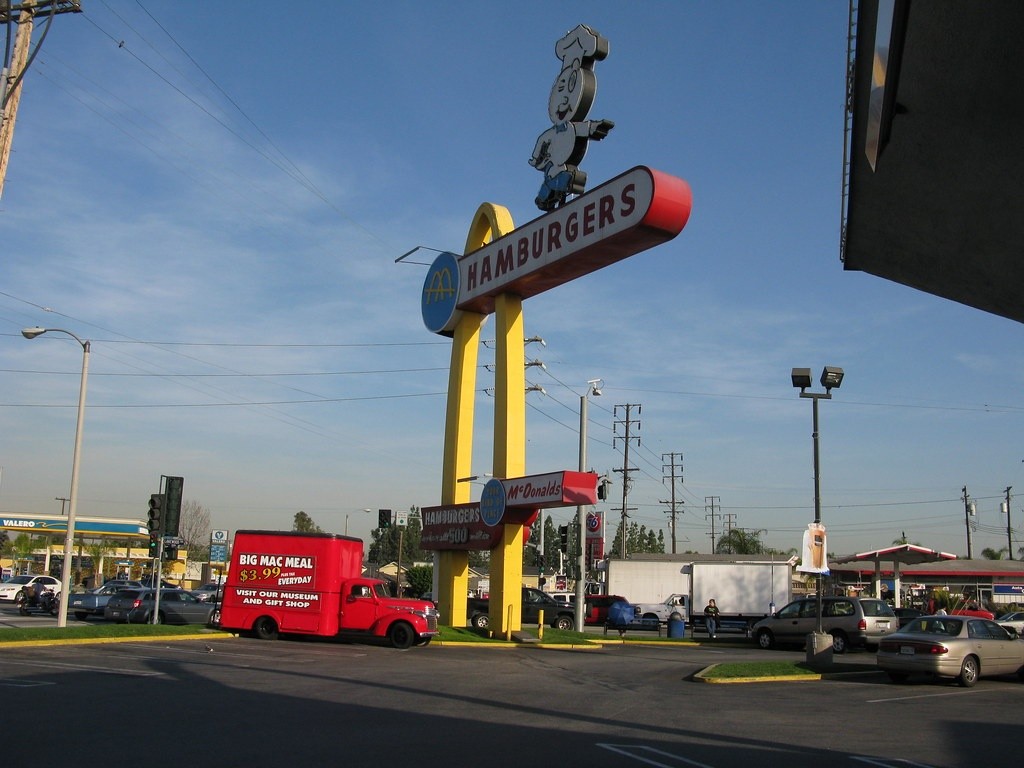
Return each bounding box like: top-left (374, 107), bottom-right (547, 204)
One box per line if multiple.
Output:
top-left (18, 586), bottom-right (61, 617)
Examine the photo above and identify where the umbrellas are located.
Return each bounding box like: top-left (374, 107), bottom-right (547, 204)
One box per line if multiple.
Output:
top-left (608, 601), bottom-right (634, 625)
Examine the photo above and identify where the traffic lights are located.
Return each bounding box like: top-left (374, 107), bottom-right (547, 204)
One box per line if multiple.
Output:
top-left (147, 532), bottom-right (159, 557)
top-left (147, 493), bottom-right (166, 529)
top-left (537, 554), bottom-right (546, 573)
top-left (379, 510), bottom-right (391, 528)
top-left (538, 578), bottom-right (546, 585)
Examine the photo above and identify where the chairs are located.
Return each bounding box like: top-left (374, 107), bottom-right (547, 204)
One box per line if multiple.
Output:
top-left (165, 594), bottom-right (179, 602)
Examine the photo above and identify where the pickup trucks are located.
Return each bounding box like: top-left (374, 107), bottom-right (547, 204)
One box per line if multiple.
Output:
top-left (68, 580), bottom-right (155, 620)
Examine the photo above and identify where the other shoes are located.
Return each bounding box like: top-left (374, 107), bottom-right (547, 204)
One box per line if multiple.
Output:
top-left (621, 633), bottom-right (626, 637)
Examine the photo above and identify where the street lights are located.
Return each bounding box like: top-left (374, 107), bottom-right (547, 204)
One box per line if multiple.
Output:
top-left (344, 508), bottom-right (372, 536)
top-left (19, 326), bottom-right (92, 627)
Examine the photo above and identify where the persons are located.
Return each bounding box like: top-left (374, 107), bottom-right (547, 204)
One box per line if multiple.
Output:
top-left (617, 624), bottom-right (626, 638)
top-left (987, 597), bottom-right (999, 620)
top-left (932, 605), bottom-right (947, 633)
top-left (32, 578), bottom-right (54, 610)
top-left (704, 599), bottom-right (720, 639)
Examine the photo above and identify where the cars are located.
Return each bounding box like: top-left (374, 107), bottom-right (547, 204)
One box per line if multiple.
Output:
top-left (947, 603), bottom-right (995, 621)
top-left (190, 583), bottom-right (224, 603)
top-left (0, 575), bottom-right (62, 603)
top-left (878, 607), bottom-right (955, 628)
top-left (987, 611), bottom-right (1024, 635)
top-left (879, 615), bottom-right (1024, 688)
top-left (104, 590), bottom-right (222, 625)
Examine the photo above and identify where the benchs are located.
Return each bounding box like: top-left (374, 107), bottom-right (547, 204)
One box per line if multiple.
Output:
top-left (690, 619), bottom-right (749, 642)
top-left (603, 618), bottom-right (662, 637)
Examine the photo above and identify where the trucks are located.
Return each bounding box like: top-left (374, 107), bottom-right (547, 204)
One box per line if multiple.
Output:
top-left (583, 559), bottom-right (689, 606)
top-left (635, 555), bottom-right (770, 628)
top-left (219, 529), bottom-right (441, 649)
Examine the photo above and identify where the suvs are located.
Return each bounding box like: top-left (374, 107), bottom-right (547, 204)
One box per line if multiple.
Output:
top-left (751, 595), bottom-right (898, 654)
top-left (465, 587), bottom-right (587, 631)
top-left (565, 595), bottom-right (630, 623)
top-left (531, 592), bottom-right (576, 607)
